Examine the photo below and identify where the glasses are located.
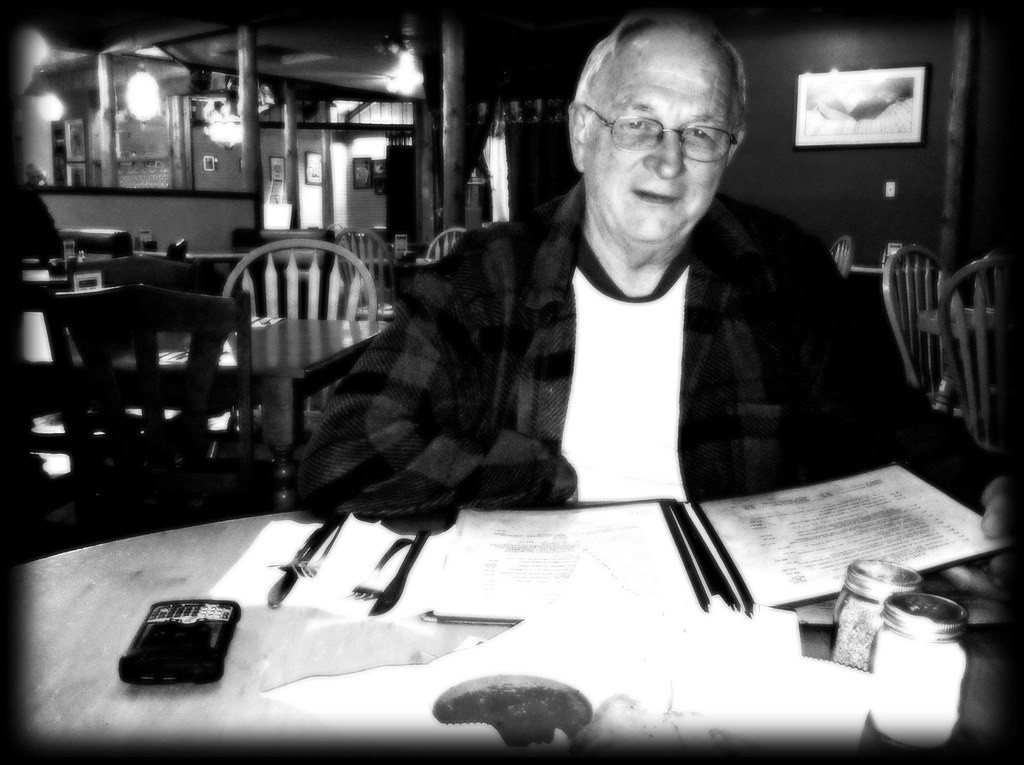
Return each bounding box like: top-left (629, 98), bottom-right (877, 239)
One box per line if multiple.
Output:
top-left (582, 104), bottom-right (737, 163)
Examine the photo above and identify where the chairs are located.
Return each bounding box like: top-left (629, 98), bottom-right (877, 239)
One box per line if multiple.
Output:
top-left (425, 224), bottom-right (468, 260)
top-left (223, 243), bottom-right (383, 433)
top-left (883, 242), bottom-right (967, 416)
top-left (57, 226), bottom-right (133, 257)
top-left (938, 252), bottom-right (1021, 456)
top-left (60, 254), bottom-right (227, 297)
top-left (825, 234), bottom-right (856, 281)
top-left (35, 274), bottom-right (298, 541)
top-left (335, 225), bottom-right (398, 320)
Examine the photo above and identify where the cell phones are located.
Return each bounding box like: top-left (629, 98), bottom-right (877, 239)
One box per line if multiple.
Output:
top-left (118, 598), bottom-right (241, 685)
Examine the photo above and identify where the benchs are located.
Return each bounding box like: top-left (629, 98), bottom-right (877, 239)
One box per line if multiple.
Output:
top-left (229, 224), bottom-right (334, 284)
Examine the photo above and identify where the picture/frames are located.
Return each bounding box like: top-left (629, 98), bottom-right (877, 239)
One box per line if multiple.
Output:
top-left (269, 155), bottom-right (286, 183)
top-left (304, 151), bottom-right (323, 186)
top-left (203, 156), bottom-right (215, 172)
top-left (791, 61), bottom-right (933, 150)
top-left (372, 159), bottom-right (389, 195)
top-left (238, 158), bottom-right (243, 173)
top-left (64, 117), bottom-right (86, 161)
top-left (66, 163), bottom-right (86, 187)
top-left (352, 157), bottom-right (373, 190)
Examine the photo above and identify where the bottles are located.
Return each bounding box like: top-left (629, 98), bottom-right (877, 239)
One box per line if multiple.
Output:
top-left (867, 591), bottom-right (969, 749)
top-left (831, 558), bottom-right (923, 671)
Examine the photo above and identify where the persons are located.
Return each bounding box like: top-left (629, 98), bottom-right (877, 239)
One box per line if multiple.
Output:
top-left (299, 13), bottom-right (1023, 602)
top-left (24, 162), bottom-right (50, 187)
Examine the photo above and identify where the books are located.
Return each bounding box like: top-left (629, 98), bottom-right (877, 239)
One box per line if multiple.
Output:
top-left (414, 460), bottom-right (1018, 641)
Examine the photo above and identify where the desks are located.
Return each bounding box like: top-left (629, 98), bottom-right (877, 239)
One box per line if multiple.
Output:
top-left (849, 261), bottom-right (934, 277)
top-left (135, 248), bottom-right (246, 268)
top-left (364, 253), bottom-right (436, 298)
top-left (19, 310), bottom-right (389, 512)
top-left (18, 489), bottom-right (938, 745)
top-left (914, 305), bottom-right (996, 339)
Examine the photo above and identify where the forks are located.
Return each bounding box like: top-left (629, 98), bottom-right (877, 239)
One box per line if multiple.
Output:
top-left (345, 539), bottom-right (412, 602)
top-left (280, 509), bottom-right (352, 578)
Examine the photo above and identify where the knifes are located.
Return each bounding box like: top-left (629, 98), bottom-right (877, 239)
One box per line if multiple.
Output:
top-left (369, 529), bottom-right (432, 616)
top-left (266, 512), bottom-right (344, 609)
